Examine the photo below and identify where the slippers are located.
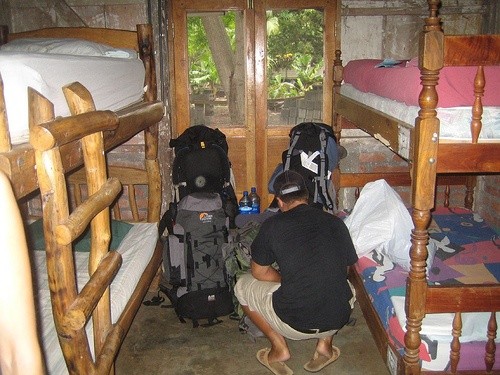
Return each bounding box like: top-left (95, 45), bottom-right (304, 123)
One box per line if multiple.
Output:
top-left (256, 348), bottom-right (293, 375)
top-left (304, 345), bottom-right (341, 372)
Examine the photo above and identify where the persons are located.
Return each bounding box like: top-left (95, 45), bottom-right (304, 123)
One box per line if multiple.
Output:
top-left (235, 170), bottom-right (358, 374)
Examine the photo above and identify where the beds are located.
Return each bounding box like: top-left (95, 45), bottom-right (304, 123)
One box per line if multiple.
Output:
top-left (0, 23), bottom-right (166, 375)
top-left (331, 0), bottom-right (500, 375)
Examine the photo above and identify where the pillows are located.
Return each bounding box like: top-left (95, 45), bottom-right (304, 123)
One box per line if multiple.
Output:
top-left (27, 218), bottom-right (135, 253)
top-left (343, 178), bottom-right (439, 278)
top-left (0, 36), bottom-right (114, 57)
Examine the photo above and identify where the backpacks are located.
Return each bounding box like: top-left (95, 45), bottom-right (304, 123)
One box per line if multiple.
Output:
top-left (157, 193), bottom-right (233, 325)
top-left (284, 122), bottom-right (337, 215)
top-left (169, 125), bottom-right (232, 203)
top-left (222, 224), bottom-right (286, 337)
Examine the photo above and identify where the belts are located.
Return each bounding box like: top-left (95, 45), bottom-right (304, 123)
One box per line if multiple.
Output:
top-left (292, 326), bottom-right (330, 334)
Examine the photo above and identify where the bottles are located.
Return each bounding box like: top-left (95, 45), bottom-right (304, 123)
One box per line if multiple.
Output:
top-left (238, 191), bottom-right (252, 215)
top-left (248, 187), bottom-right (260, 214)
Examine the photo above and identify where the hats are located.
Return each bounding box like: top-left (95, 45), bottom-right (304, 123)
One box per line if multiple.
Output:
top-left (273, 171), bottom-right (305, 197)
